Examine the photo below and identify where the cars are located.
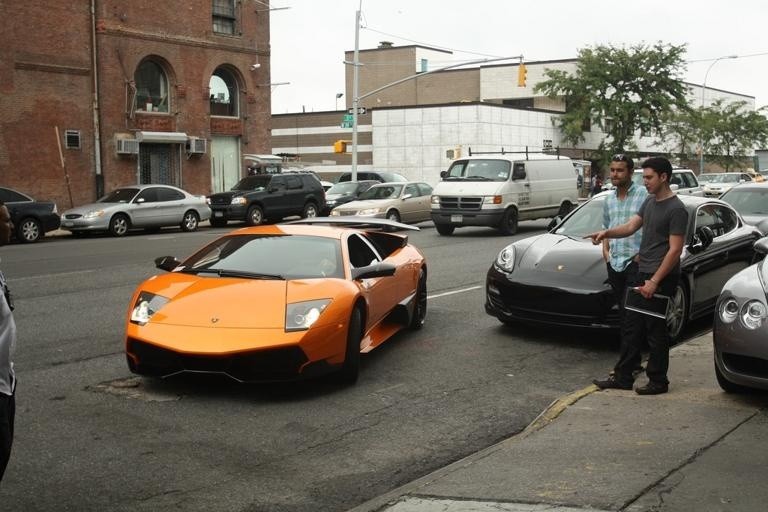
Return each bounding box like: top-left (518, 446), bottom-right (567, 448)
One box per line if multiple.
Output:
top-left (207, 161), bottom-right (436, 229)
top-left (0, 187), bottom-right (60, 245)
top-left (59, 184), bottom-right (212, 238)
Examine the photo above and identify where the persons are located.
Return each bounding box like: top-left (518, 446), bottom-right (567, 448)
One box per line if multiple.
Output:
top-left (582, 157), bottom-right (689, 395)
top-left (590, 173), bottom-right (603, 196)
top-left (575, 169), bottom-right (583, 198)
top-left (602, 154), bottom-right (648, 376)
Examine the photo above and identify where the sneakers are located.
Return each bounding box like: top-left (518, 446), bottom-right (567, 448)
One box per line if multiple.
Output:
top-left (590, 372), bottom-right (634, 392)
top-left (635, 379), bottom-right (670, 397)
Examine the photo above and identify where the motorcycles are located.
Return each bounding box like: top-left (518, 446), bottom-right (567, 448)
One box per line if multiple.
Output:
top-left (118, 214), bottom-right (430, 392)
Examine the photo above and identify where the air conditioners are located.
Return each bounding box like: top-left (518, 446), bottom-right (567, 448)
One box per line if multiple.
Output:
top-left (117, 139), bottom-right (139, 154)
top-left (183, 137), bottom-right (207, 153)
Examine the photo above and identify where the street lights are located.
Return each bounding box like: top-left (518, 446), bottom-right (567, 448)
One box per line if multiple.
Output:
top-left (334, 93), bottom-right (343, 110)
top-left (698, 55), bottom-right (738, 175)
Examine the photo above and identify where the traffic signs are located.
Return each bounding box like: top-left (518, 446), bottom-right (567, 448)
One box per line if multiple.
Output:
top-left (340, 114), bottom-right (353, 121)
top-left (340, 122), bottom-right (353, 128)
top-left (348, 108), bottom-right (366, 114)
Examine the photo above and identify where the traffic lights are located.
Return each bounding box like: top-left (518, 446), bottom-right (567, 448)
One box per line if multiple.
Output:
top-left (517, 64), bottom-right (528, 87)
top-left (334, 140), bottom-right (347, 154)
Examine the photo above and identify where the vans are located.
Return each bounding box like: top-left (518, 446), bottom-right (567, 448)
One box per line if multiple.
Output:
top-left (427, 144), bottom-right (579, 237)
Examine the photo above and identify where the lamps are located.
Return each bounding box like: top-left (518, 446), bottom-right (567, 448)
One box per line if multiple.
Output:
top-left (249, 63), bottom-right (261, 71)
